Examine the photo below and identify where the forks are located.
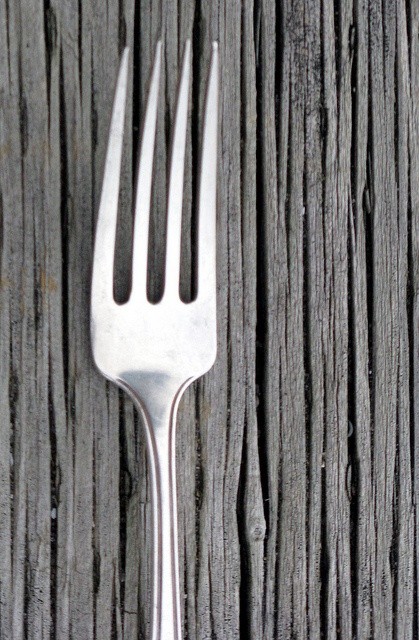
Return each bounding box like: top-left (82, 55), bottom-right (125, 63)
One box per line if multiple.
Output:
top-left (88, 40), bottom-right (220, 639)
